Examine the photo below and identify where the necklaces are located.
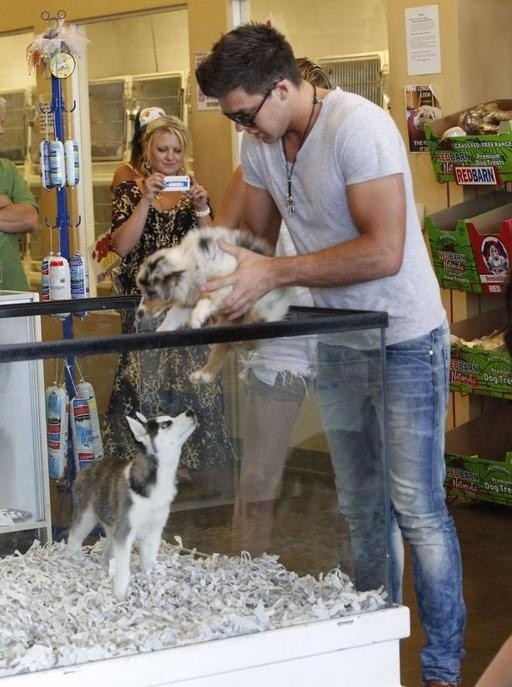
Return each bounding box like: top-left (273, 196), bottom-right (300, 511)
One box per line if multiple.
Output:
top-left (153, 193), bottom-right (170, 202)
top-left (280, 82), bottom-right (317, 214)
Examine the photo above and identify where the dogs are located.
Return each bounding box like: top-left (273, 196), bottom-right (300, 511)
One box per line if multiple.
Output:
top-left (135, 225), bottom-right (291, 384)
top-left (67, 408), bottom-right (199, 602)
top-left (482, 235), bottom-right (508, 269)
top-left (413, 104), bottom-right (443, 131)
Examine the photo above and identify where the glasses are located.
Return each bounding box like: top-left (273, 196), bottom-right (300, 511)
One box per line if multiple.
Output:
top-left (222, 86), bottom-right (276, 128)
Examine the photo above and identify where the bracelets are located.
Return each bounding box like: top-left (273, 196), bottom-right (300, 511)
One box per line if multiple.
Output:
top-left (194, 207), bottom-right (214, 217)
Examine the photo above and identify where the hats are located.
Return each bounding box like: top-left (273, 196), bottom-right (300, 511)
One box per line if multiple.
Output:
top-left (132, 106), bottom-right (168, 132)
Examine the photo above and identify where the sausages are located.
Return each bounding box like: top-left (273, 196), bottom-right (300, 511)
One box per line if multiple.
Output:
top-left (40, 134), bottom-right (104, 483)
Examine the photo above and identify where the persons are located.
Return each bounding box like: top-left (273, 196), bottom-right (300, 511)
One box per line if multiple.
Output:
top-left (110, 114), bottom-right (237, 485)
top-left (195, 19), bottom-right (469, 685)
top-left (113, 106), bottom-right (167, 195)
top-left (0, 98), bottom-right (41, 291)
top-left (212, 53), bottom-right (354, 584)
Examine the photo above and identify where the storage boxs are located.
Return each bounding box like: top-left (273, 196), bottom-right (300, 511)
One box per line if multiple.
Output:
top-left (422, 99), bottom-right (511, 508)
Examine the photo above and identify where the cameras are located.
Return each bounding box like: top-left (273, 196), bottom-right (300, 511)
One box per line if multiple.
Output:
top-left (162, 175), bottom-right (191, 193)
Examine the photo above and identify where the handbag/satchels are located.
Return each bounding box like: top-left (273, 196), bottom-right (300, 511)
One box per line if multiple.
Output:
top-left (93, 226), bottom-right (124, 300)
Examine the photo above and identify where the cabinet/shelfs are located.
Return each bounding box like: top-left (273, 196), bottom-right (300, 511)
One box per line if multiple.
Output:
top-left (0, 289), bottom-right (53, 549)
